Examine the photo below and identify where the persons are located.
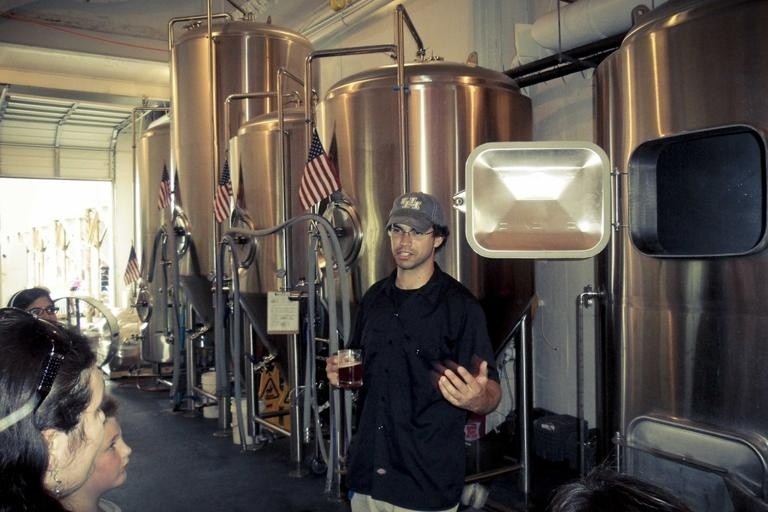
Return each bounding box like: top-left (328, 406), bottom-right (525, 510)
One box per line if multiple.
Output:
top-left (58, 388), bottom-right (135, 512)
top-left (325, 191), bottom-right (503, 512)
top-left (0, 305), bottom-right (109, 512)
top-left (5, 284), bottom-right (60, 323)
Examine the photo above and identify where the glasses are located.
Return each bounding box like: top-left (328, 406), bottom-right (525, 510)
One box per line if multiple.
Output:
top-left (26, 306), bottom-right (59, 316)
top-left (0, 306), bottom-right (68, 434)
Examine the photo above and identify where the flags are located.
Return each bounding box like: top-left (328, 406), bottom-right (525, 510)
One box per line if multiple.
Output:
top-left (172, 166), bottom-right (183, 209)
top-left (139, 246), bottom-right (147, 280)
top-left (235, 153), bottom-right (247, 210)
top-left (212, 158), bottom-right (235, 224)
top-left (123, 243), bottom-right (141, 287)
top-left (296, 124), bottom-right (343, 213)
top-left (155, 162), bottom-right (172, 212)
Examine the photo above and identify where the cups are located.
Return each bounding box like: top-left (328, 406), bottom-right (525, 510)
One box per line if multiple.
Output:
top-left (337, 348), bottom-right (364, 391)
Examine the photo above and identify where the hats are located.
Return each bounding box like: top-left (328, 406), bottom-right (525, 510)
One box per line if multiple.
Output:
top-left (385, 191), bottom-right (446, 233)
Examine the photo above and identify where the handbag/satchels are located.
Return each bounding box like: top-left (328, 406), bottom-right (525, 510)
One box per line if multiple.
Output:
top-left (346, 426), bottom-right (392, 484)
